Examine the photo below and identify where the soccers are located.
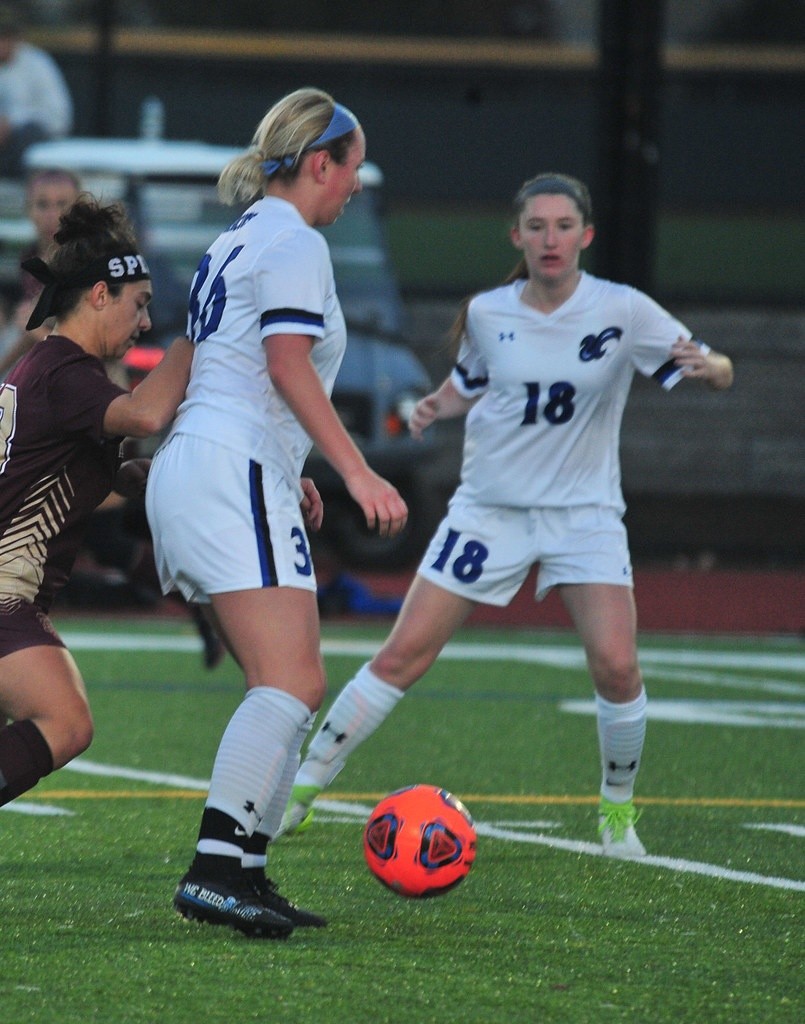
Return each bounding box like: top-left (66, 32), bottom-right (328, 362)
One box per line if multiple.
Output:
top-left (364, 783), bottom-right (477, 900)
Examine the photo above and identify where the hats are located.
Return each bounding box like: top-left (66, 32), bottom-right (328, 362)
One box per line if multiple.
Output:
top-left (25, 239), bottom-right (149, 332)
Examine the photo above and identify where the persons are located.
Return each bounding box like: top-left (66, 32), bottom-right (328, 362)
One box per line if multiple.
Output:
top-left (145, 89), bottom-right (408, 942)
top-left (269, 172), bottom-right (733, 857)
top-left (1, 168), bottom-right (227, 809)
top-left (0, 23), bottom-right (76, 184)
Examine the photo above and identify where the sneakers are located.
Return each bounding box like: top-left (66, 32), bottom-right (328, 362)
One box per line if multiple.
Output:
top-left (266, 803), bottom-right (315, 841)
top-left (237, 877), bottom-right (328, 929)
top-left (172, 861), bottom-right (294, 940)
top-left (596, 796), bottom-right (647, 859)
